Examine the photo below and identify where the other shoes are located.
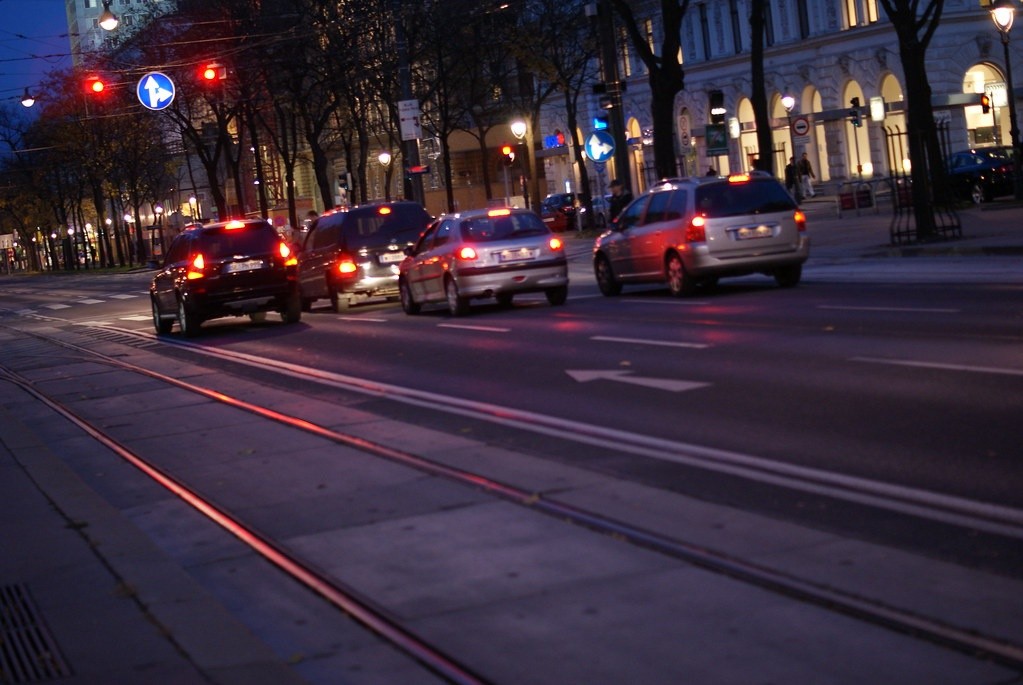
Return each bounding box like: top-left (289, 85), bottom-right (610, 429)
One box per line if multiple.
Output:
top-left (800, 196), bottom-right (805, 199)
top-left (811, 194), bottom-right (817, 198)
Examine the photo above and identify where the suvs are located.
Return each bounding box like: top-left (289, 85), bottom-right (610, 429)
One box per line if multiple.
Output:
top-left (294, 197), bottom-right (437, 314)
top-left (150, 213), bottom-right (304, 339)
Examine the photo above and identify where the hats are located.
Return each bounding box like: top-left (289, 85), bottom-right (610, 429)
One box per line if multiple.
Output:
top-left (608, 179), bottom-right (624, 188)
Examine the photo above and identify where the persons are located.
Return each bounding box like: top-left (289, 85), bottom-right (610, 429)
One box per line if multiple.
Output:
top-left (607, 179), bottom-right (633, 225)
top-left (785, 153), bottom-right (816, 205)
top-left (79, 246), bottom-right (96, 264)
top-left (308, 211), bottom-right (318, 222)
top-left (706, 166), bottom-right (716, 176)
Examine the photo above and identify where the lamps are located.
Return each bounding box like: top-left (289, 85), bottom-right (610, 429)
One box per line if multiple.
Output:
top-left (701, 47), bottom-right (896, 96)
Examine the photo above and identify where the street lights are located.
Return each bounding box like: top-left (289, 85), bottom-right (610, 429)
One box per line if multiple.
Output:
top-left (986, 0), bottom-right (1023, 156)
top-left (780, 95), bottom-right (796, 162)
top-left (511, 122), bottom-right (531, 209)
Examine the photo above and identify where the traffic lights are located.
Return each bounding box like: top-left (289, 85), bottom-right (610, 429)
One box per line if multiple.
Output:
top-left (196, 62), bottom-right (220, 84)
top-left (982, 92), bottom-right (990, 113)
top-left (87, 75), bottom-right (106, 96)
top-left (849, 97), bottom-right (862, 128)
top-left (497, 143), bottom-right (515, 159)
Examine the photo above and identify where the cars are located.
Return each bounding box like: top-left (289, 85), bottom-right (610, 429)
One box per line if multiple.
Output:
top-left (397, 206), bottom-right (570, 318)
top-left (591, 194), bottom-right (613, 228)
top-left (540, 192), bottom-right (589, 233)
top-left (921, 144), bottom-right (1017, 207)
top-left (590, 169), bottom-right (811, 299)
